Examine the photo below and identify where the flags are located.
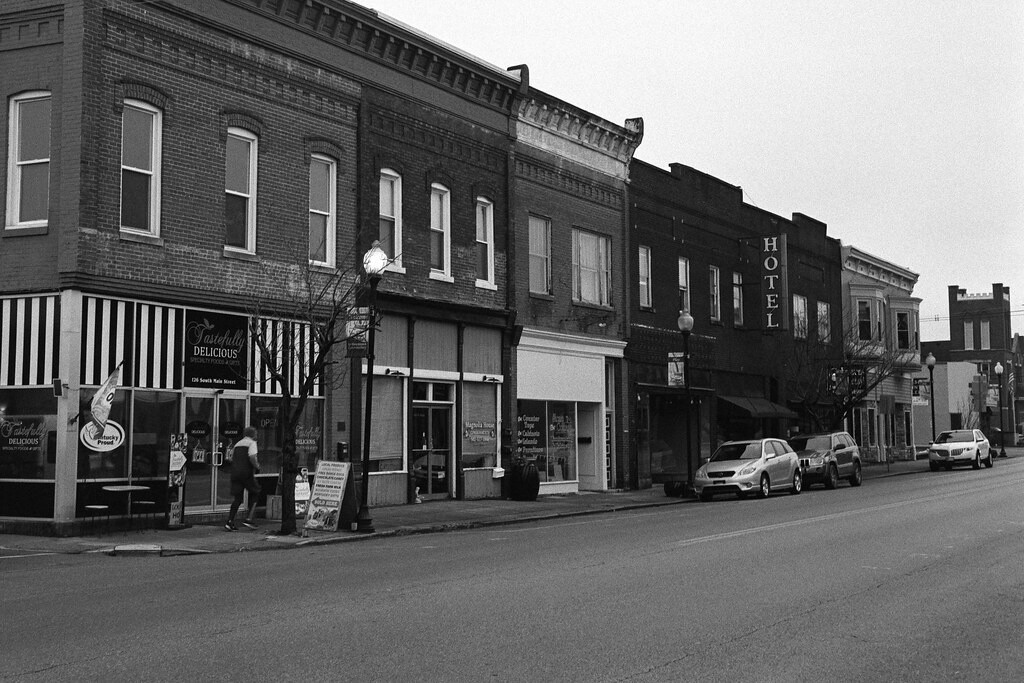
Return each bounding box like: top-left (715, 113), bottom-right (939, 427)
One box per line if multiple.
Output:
top-left (90, 368), bottom-right (119, 439)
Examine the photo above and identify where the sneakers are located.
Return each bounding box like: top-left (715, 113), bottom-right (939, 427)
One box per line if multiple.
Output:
top-left (226, 522), bottom-right (238, 531)
top-left (243, 520), bottom-right (257, 529)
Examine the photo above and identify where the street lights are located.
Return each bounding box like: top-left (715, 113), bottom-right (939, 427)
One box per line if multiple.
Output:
top-left (926, 351), bottom-right (937, 444)
top-left (996, 361), bottom-right (1008, 457)
top-left (351, 240), bottom-right (388, 534)
top-left (677, 308), bottom-right (698, 499)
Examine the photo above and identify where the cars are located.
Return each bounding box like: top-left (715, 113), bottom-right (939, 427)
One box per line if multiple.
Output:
top-left (695, 438), bottom-right (803, 499)
top-left (415, 450), bottom-right (447, 483)
top-left (929, 428), bottom-right (993, 471)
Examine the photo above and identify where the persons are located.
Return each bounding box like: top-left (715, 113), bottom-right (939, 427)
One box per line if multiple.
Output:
top-left (224, 426), bottom-right (263, 532)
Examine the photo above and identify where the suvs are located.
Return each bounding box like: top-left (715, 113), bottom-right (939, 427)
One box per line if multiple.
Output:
top-left (787, 432), bottom-right (863, 490)
